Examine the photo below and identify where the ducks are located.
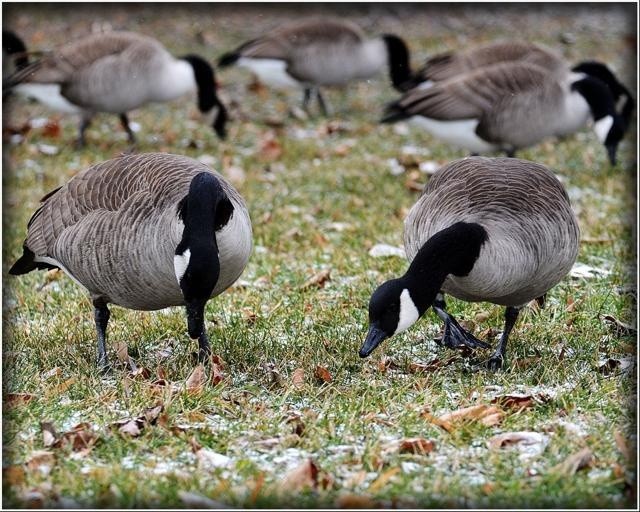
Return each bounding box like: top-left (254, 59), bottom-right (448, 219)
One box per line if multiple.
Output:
top-left (215, 19), bottom-right (415, 119)
top-left (377, 61), bottom-right (637, 166)
top-left (402, 38), bottom-right (615, 83)
top-left (356, 154), bottom-right (582, 375)
top-left (2, 31), bottom-right (230, 154)
top-left (7, 150), bottom-right (256, 378)
